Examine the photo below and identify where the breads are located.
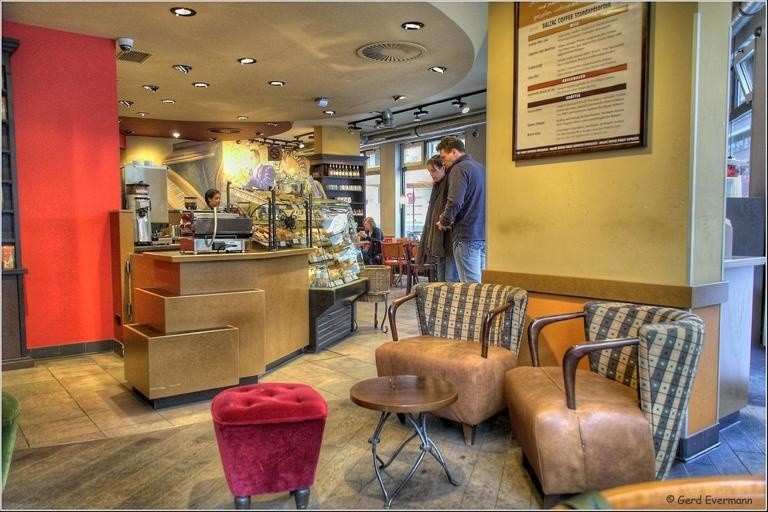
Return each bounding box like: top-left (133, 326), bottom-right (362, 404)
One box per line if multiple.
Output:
top-left (251, 189), bottom-right (355, 286)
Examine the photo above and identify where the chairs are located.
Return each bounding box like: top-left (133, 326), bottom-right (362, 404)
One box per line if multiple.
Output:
top-left (374, 280), bottom-right (529, 447)
top-left (504, 298), bottom-right (706, 497)
top-left (550, 475), bottom-right (766, 510)
top-left (381, 234), bottom-right (438, 296)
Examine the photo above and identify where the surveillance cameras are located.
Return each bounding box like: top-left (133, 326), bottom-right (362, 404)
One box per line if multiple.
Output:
top-left (472, 131), bottom-right (479, 138)
top-left (118, 38), bottom-right (134, 53)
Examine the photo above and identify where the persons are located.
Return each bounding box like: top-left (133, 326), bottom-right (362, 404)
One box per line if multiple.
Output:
top-left (436, 135), bottom-right (486, 283)
top-left (238, 147), bottom-right (274, 191)
top-left (356, 216), bottom-right (384, 265)
top-left (200, 189), bottom-right (222, 210)
top-left (415, 155), bottom-right (459, 283)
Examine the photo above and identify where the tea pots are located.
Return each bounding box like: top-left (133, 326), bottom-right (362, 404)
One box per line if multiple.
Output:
top-left (273, 173), bottom-right (309, 198)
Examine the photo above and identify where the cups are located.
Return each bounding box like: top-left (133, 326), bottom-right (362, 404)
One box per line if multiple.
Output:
top-left (326, 184), bottom-right (363, 203)
top-left (2, 246), bottom-right (15, 269)
top-left (184, 196), bottom-right (197, 210)
top-left (167, 209), bottom-right (181, 224)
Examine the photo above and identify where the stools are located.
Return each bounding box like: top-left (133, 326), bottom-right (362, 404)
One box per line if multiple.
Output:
top-left (205, 380), bottom-right (329, 510)
top-left (1, 391), bottom-right (26, 497)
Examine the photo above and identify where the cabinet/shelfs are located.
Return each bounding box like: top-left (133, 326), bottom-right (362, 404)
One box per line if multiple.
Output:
top-left (1, 35), bottom-right (38, 372)
top-left (229, 183), bottom-right (370, 352)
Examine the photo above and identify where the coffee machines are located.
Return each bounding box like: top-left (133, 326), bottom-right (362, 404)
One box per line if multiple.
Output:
top-left (125, 180), bottom-right (153, 246)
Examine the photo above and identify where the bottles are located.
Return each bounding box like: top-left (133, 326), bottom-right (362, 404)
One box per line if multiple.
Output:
top-left (154, 224), bottom-right (181, 238)
top-left (352, 208), bottom-right (363, 215)
top-left (328, 163), bottom-right (360, 178)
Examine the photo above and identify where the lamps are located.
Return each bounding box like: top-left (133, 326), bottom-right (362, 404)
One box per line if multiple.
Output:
top-left (347, 90), bottom-right (486, 139)
